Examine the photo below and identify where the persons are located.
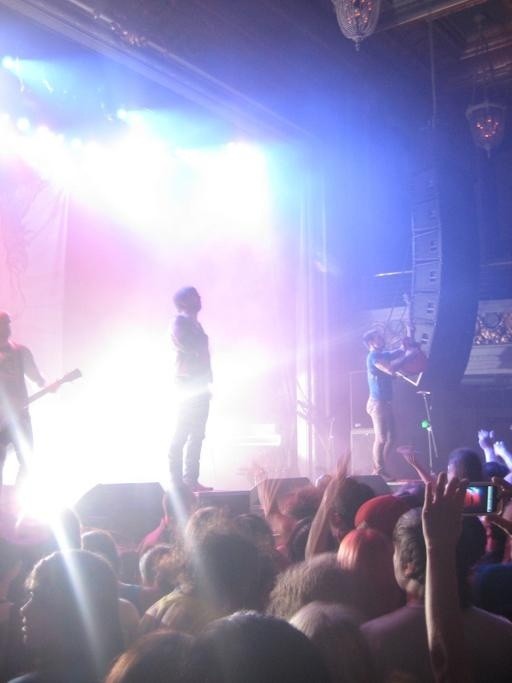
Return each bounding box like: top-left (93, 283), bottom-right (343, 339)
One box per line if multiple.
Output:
top-left (0, 310), bottom-right (58, 486)
top-left (167, 284), bottom-right (216, 494)
top-left (1, 427), bottom-right (511, 682)
top-left (361, 327), bottom-right (421, 483)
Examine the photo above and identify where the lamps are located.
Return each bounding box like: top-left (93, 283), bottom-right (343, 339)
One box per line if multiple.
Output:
top-left (329, 0), bottom-right (381, 53)
top-left (464, 0), bottom-right (508, 158)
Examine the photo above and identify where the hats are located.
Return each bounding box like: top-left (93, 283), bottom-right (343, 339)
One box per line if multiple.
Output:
top-left (353, 493), bottom-right (410, 541)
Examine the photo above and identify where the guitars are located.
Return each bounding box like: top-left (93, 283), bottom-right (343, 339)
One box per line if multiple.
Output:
top-left (401, 292), bottom-right (429, 375)
top-left (0, 368), bottom-right (83, 430)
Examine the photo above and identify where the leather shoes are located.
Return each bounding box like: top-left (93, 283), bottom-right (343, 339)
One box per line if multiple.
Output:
top-left (184, 480), bottom-right (212, 493)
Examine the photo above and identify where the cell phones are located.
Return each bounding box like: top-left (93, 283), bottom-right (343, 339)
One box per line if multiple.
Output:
top-left (456, 482), bottom-right (503, 518)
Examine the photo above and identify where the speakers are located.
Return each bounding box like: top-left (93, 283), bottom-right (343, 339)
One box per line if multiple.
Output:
top-left (194, 491), bottom-right (253, 516)
top-left (252, 476), bottom-right (317, 519)
top-left (349, 369), bottom-right (373, 430)
top-left (349, 430), bottom-right (377, 475)
top-left (67, 483), bottom-right (177, 545)
top-left (350, 474), bottom-right (397, 496)
top-left (394, 160), bottom-right (481, 393)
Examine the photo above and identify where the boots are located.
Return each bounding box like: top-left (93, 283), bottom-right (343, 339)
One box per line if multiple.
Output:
top-left (372, 466), bottom-right (396, 482)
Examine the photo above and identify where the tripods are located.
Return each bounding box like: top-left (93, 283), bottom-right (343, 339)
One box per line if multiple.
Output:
top-left (421, 395), bottom-right (441, 477)
